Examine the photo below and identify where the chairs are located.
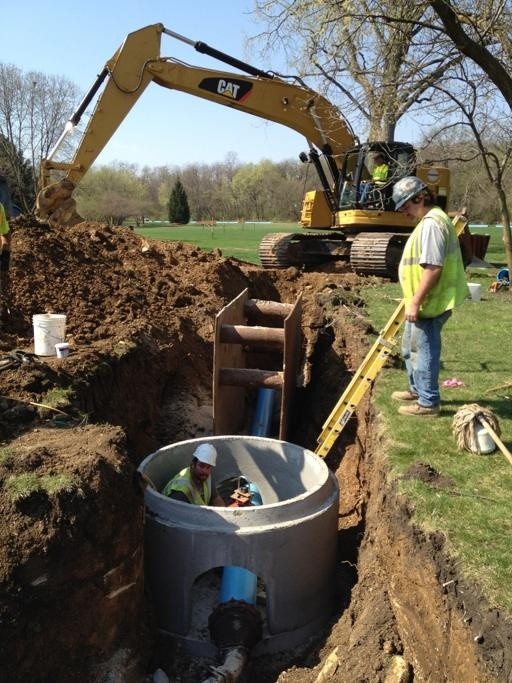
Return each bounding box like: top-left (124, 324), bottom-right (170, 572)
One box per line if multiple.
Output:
top-left (370, 164), bottom-right (399, 197)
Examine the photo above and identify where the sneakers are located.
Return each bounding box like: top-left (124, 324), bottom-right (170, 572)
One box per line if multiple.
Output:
top-left (393, 392), bottom-right (419, 401)
top-left (399, 404), bottom-right (440, 417)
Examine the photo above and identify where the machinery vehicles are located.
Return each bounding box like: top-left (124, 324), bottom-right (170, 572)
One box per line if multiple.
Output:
top-left (28, 22), bottom-right (491, 284)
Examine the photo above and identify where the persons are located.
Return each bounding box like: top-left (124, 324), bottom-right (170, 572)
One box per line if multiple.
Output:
top-left (354, 152), bottom-right (390, 204)
top-left (159, 444), bottom-right (240, 508)
top-left (390, 176), bottom-right (470, 417)
top-left (0, 201), bottom-right (10, 273)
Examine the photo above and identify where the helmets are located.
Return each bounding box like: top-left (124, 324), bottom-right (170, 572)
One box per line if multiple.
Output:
top-left (393, 176), bottom-right (428, 211)
top-left (193, 444), bottom-right (217, 467)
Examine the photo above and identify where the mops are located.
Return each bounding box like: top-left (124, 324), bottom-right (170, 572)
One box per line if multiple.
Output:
top-left (451, 403), bottom-right (512, 465)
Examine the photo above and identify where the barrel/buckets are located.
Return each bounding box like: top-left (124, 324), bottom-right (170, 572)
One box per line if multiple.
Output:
top-left (496, 269), bottom-right (509, 285)
top-left (32, 314), bottom-right (67, 356)
top-left (55, 342), bottom-right (70, 359)
top-left (465, 283), bottom-right (482, 301)
